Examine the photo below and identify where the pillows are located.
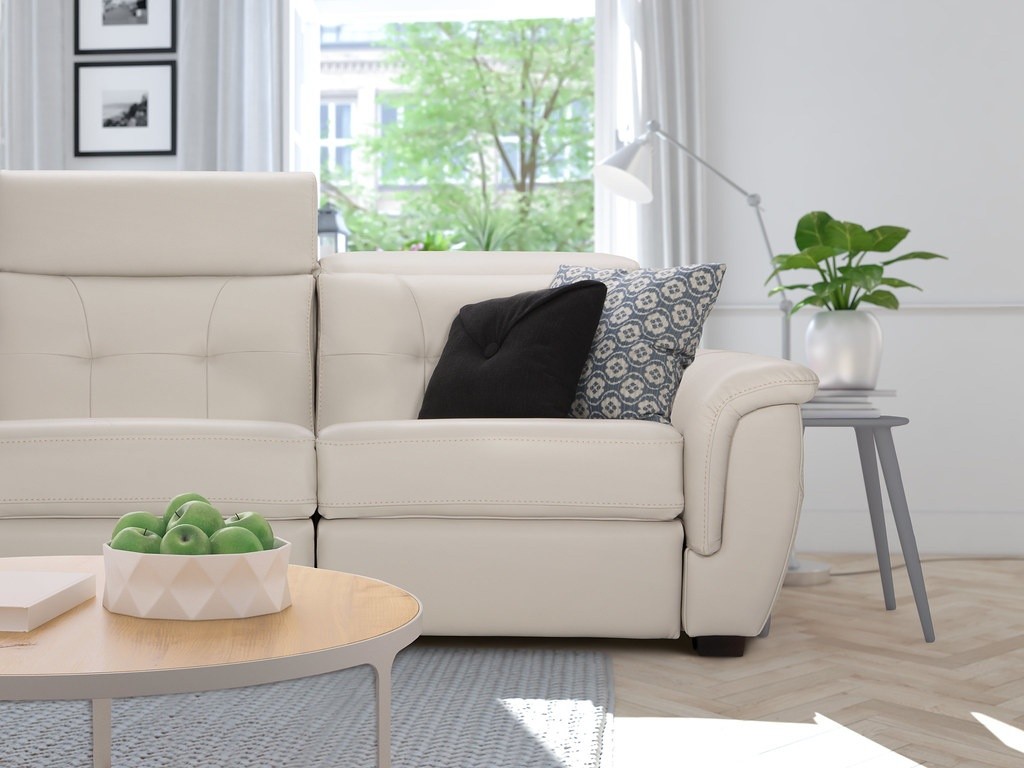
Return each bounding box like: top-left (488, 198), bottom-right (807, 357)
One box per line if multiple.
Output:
top-left (418, 280), bottom-right (607, 420)
top-left (549, 262), bottom-right (727, 426)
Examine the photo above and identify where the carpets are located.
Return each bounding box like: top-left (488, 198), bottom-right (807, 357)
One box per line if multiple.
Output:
top-left (0, 642), bottom-right (617, 768)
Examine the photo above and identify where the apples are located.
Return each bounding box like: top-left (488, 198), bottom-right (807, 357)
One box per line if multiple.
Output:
top-left (166, 500), bottom-right (226, 538)
top-left (209, 526), bottom-right (263, 554)
top-left (112, 511), bottom-right (165, 538)
top-left (110, 526), bottom-right (162, 554)
top-left (162, 492), bottom-right (210, 525)
top-left (159, 523), bottom-right (212, 555)
top-left (225, 511), bottom-right (274, 551)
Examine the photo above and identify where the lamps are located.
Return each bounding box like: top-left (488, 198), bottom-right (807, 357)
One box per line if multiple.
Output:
top-left (593, 121), bottom-right (831, 587)
top-left (318, 200), bottom-right (348, 261)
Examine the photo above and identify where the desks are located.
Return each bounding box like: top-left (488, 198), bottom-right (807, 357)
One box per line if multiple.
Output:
top-left (0, 556), bottom-right (423, 768)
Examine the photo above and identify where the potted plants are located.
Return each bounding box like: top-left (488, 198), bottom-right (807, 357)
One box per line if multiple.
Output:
top-left (762, 210), bottom-right (949, 391)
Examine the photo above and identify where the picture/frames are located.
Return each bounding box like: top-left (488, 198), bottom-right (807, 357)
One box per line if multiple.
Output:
top-left (73, 0), bottom-right (176, 55)
top-left (73, 60), bottom-right (177, 157)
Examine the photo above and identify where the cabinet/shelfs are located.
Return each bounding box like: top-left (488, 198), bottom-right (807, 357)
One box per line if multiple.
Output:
top-left (802, 415), bottom-right (936, 644)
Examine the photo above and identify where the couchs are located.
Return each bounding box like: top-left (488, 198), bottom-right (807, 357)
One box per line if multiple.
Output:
top-left (1, 169), bottom-right (821, 660)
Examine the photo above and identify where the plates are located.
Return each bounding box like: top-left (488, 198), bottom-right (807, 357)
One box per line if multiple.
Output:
top-left (101, 536), bottom-right (293, 622)
